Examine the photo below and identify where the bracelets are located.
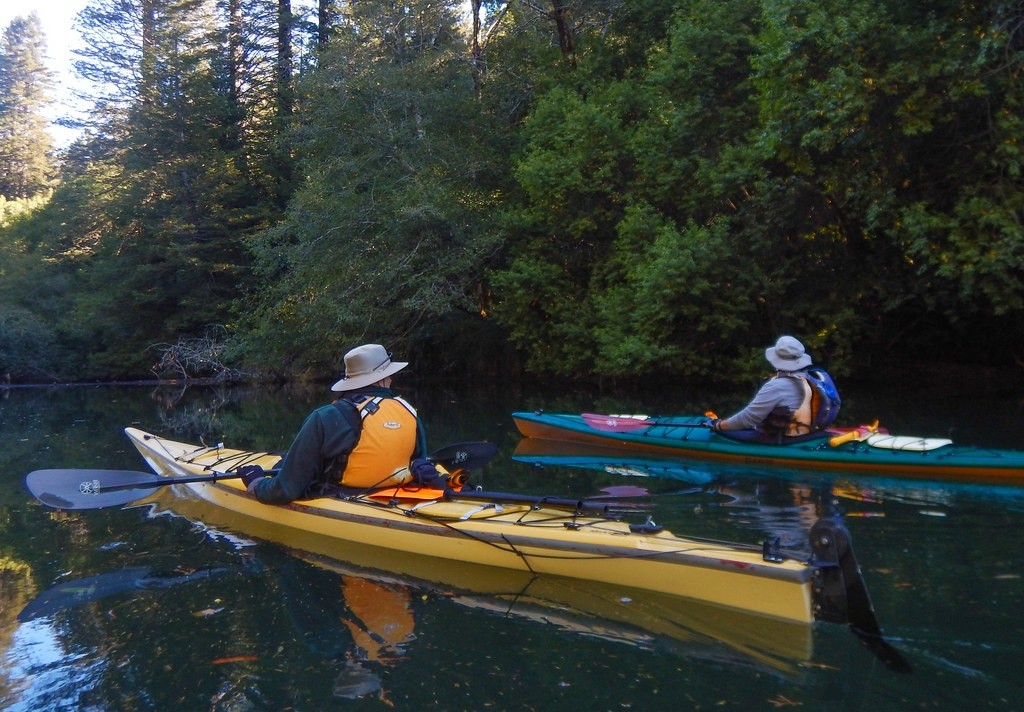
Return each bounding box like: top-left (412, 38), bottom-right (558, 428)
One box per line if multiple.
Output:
top-left (718, 419), bottom-right (721, 430)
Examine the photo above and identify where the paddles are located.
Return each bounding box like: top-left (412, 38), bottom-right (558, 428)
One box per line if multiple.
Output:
top-left (579, 412), bottom-right (711, 432)
top-left (369, 487), bottom-right (610, 513)
top-left (25, 441), bottom-right (500, 510)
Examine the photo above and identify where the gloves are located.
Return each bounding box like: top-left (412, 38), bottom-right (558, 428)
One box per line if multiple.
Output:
top-left (237, 465), bottom-right (265, 492)
top-left (701, 418), bottom-right (720, 432)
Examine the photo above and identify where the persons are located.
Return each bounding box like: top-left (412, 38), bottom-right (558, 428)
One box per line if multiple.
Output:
top-left (237, 343), bottom-right (427, 505)
top-left (711, 336), bottom-right (841, 440)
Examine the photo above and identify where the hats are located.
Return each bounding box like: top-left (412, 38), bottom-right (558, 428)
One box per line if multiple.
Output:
top-left (765, 336), bottom-right (812, 371)
top-left (330, 345), bottom-right (408, 392)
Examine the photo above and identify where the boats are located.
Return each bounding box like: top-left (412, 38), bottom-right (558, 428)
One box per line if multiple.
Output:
top-left (512, 408), bottom-right (1024, 488)
top-left (124, 426), bottom-right (883, 649)
